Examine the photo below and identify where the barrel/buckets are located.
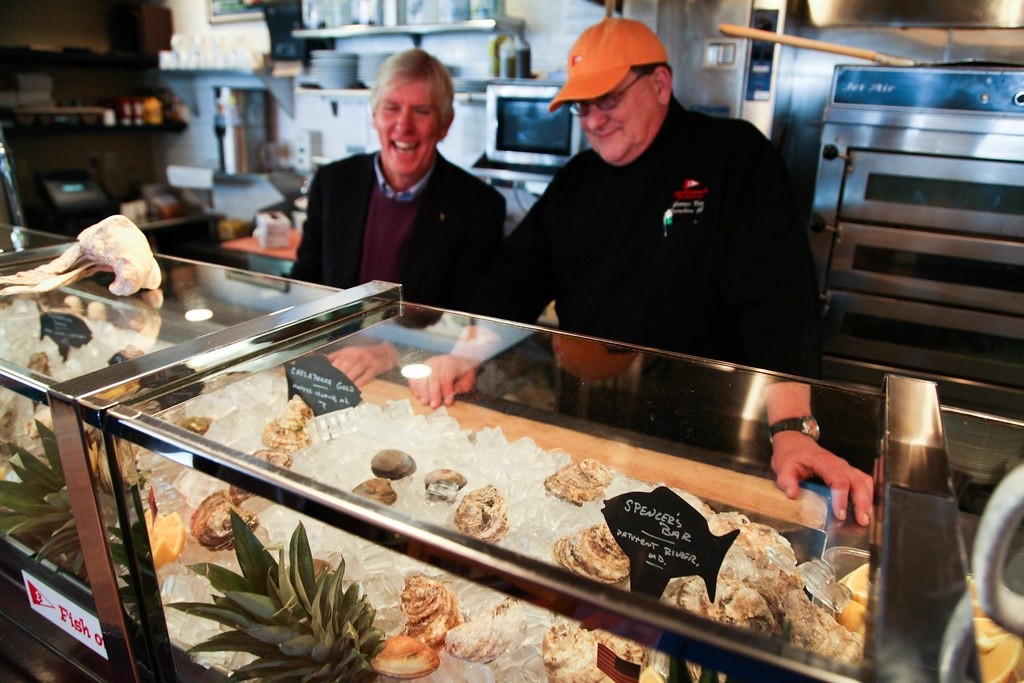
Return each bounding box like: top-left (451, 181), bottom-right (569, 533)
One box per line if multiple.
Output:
top-left (210, 81), bottom-right (269, 174)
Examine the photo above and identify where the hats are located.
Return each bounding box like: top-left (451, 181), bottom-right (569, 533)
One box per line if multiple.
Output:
top-left (548, 16), bottom-right (668, 112)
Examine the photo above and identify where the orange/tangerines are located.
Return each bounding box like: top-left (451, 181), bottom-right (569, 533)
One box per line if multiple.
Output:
top-left (144, 508), bottom-right (186, 570)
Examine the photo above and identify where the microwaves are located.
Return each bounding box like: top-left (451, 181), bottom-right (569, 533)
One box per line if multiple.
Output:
top-left (483, 84), bottom-right (587, 173)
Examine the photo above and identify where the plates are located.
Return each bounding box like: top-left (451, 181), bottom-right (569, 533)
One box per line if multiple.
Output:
top-left (304, 47), bottom-right (491, 94)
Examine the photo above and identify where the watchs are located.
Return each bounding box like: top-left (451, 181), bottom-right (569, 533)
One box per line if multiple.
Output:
top-left (768, 413), bottom-right (822, 444)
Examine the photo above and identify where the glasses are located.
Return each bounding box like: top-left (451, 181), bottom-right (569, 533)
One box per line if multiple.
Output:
top-left (569, 72), bottom-right (645, 117)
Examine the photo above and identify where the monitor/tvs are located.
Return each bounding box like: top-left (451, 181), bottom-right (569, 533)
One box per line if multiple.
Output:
top-left (485, 84), bottom-right (582, 168)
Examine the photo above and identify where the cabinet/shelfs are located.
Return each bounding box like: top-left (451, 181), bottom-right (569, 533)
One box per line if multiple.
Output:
top-left (290, 1), bottom-right (530, 108)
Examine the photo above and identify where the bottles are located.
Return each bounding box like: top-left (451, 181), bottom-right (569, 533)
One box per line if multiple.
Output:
top-left (119, 30), bottom-right (266, 127)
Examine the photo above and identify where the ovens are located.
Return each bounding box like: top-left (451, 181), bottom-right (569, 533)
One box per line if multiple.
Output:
top-left (789, 63), bottom-right (1024, 417)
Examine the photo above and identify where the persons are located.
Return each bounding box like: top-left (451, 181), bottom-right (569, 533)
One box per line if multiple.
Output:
top-left (405, 17), bottom-right (879, 528)
top-left (282, 46), bottom-right (513, 393)
